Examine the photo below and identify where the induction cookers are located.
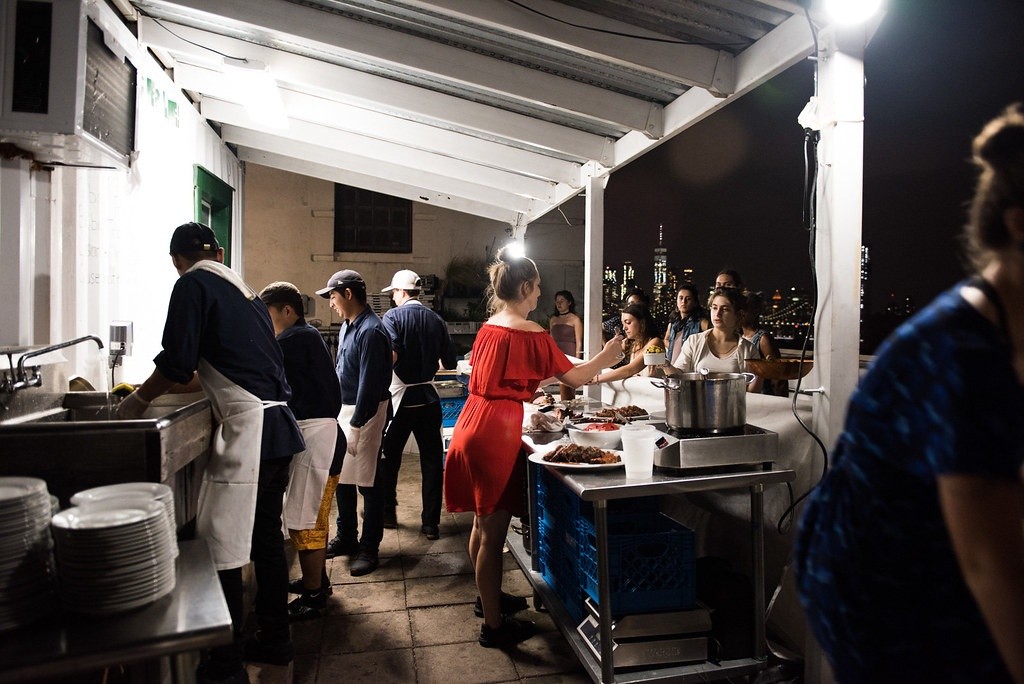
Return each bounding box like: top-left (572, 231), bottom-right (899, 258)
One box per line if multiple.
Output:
top-left (644, 421), bottom-right (779, 477)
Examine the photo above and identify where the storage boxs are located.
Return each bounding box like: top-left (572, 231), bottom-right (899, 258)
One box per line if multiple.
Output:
top-left (437, 396), bottom-right (469, 472)
top-left (533, 463), bottom-right (699, 627)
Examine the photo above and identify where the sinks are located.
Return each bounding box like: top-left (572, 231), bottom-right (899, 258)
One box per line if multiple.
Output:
top-left (1, 391), bottom-right (214, 485)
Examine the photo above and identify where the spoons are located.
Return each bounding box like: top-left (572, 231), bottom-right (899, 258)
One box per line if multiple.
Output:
top-left (614, 325), bottom-right (625, 360)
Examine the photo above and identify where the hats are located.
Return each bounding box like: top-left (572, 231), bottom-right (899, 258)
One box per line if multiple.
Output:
top-left (169, 221), bottom-right (219, 254)
top-left (381, 270), bottom-right (421, 293)
top-left (315, 270), bottom-right (363, 299)
top-left (259, 280), bottom-right (300, 303)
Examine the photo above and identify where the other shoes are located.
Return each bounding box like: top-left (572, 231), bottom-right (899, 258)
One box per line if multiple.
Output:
top-left (285, 578), bottom-right (330, 609)
top-left (474, 591), bottom-right (529, 617)
top-left (327, 538), bottom-right (358, 558)
top-left (421, 524), bottom-right (439, 539)
top-left (360, 511), bottom-right (398, 527)
top-left (480, 617), bottom-right (534, 645)
top-left (350, 551), bottom-right (379, 576)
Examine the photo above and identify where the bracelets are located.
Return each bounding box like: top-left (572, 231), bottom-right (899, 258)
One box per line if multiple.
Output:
top-left (595, 374), bottom-right (601, 385)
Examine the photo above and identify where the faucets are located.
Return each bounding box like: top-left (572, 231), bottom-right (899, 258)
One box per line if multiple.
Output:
top-left (1, 335), bottom-right (105, 396)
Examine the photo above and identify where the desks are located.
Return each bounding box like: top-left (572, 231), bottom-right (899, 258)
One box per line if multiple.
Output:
top-left (523, 392), bottom-right (622, 420)
top-left (522, 420), bottom-right (802, 684)
top-left (0, 533), bottom-right (239, 684)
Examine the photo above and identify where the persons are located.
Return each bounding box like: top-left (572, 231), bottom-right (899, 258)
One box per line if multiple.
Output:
top-left (113, 223), bottom-right (306, 679)
top-left (586, 268), bottom-right (792, 398)
top-left (790, 113), bottom-right (1024, 684)
top-left (442, 245), bottom-right (624, 648)
top-left (313, 270), bottom-right (393, 575)
top-left (548, 289), bottom-right (583, 358)
top-left (256, 282), bottom-right (349, 623)
top-left (377, 269), bottom-right (458, 541)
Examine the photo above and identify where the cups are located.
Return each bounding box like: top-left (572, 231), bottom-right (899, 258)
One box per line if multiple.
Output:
top-left (559, 382), bottom-right (576, 401)
top-left (620, 424), bottom-right (656, 480)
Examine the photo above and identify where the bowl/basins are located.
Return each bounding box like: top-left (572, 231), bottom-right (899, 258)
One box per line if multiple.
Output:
top-left (643, 353), bottom-right (665, 365)
top-left (568, 423), bottom-right (624, 450)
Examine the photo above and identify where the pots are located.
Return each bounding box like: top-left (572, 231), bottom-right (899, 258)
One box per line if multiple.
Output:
top-left (650, 372), bottom-right (756, 433)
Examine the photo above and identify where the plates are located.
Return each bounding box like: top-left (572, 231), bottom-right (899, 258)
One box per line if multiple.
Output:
top-left (593, 414), bottom-right (650, 421)
top-left (527, 449), bottom-right (625, 469)
top-left (0, 476), bottom-right (179, 632)
